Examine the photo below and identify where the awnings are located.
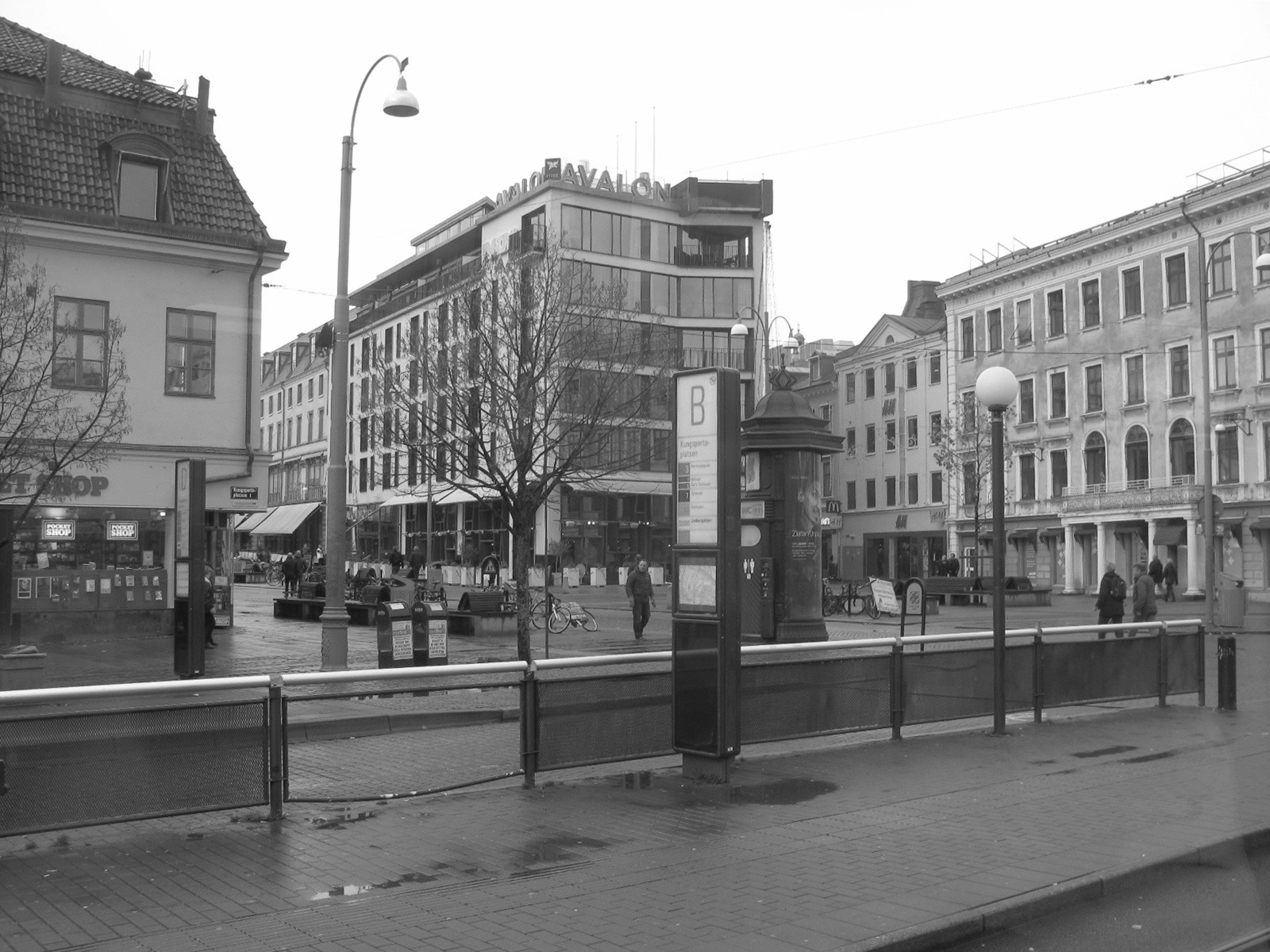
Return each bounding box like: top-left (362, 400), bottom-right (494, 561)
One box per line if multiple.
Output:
top-left (379, 483), bottom-right (507, 505)
top-left (561, 477), bottom-right (674, 497)
top-left (1152, 521), bottom-right (1187, 545)
top-left (978, 523), bottom-right (1148, 549)
top-left (234, 502), bottom-right (322, 536)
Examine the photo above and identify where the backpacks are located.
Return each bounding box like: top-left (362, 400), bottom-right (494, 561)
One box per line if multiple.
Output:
top-left (1103, 572), bottom-right (1127, 599)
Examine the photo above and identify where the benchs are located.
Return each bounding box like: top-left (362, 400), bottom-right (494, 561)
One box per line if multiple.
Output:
top-left (273, 583), bottom-right (518, 636)
top-left (924, 576), bottom-right (1053, 608)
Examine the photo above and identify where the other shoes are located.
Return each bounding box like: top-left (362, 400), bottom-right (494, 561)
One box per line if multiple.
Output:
top-left (636, 636), bottom-right (647, 640)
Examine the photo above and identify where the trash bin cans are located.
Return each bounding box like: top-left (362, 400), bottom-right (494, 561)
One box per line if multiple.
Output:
top-left (376, 601), bottom-right (416, 669)
top-left (1216, 572), bottom-right (1245, 627)
top-left (411, 599), bottom-right (450, 666)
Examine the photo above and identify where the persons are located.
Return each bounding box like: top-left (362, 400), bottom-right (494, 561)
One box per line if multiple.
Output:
top-left (388, 545), bottom-right (404, 575)
top-left (233, 543), bottom-right (325, 598)
top-left (625, 552), bottom-right (656, 641)
top-left (408, 546), bottom-right (426, 578)
top-left (204, 566), bottom-right (218, 649)
top-left (1094, 554), bottom-right (1179, 640)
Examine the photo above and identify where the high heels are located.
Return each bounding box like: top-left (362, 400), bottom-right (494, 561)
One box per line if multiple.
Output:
top-left (205, 637), bottom-right (218, 645)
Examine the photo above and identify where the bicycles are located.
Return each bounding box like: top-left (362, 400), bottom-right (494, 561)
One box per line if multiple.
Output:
top-left (264, 555), bottom-right (328, 588)
top-left (822, 576), bottom-right (898, 619)
top-left (484, 580), bottom-right (599, 634)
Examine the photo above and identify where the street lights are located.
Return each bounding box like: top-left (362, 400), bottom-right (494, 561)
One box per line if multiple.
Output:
top-left (974, 366), bottom-right (1020, 735)
top-left (730, 306), bottom-right (800, 397)
top-left (408, 438), bottom-right (445, 589)
top-left (323, 51), bottom-right (422, 670)
top-left (1196, 231), bottom-right (1270, 634)
